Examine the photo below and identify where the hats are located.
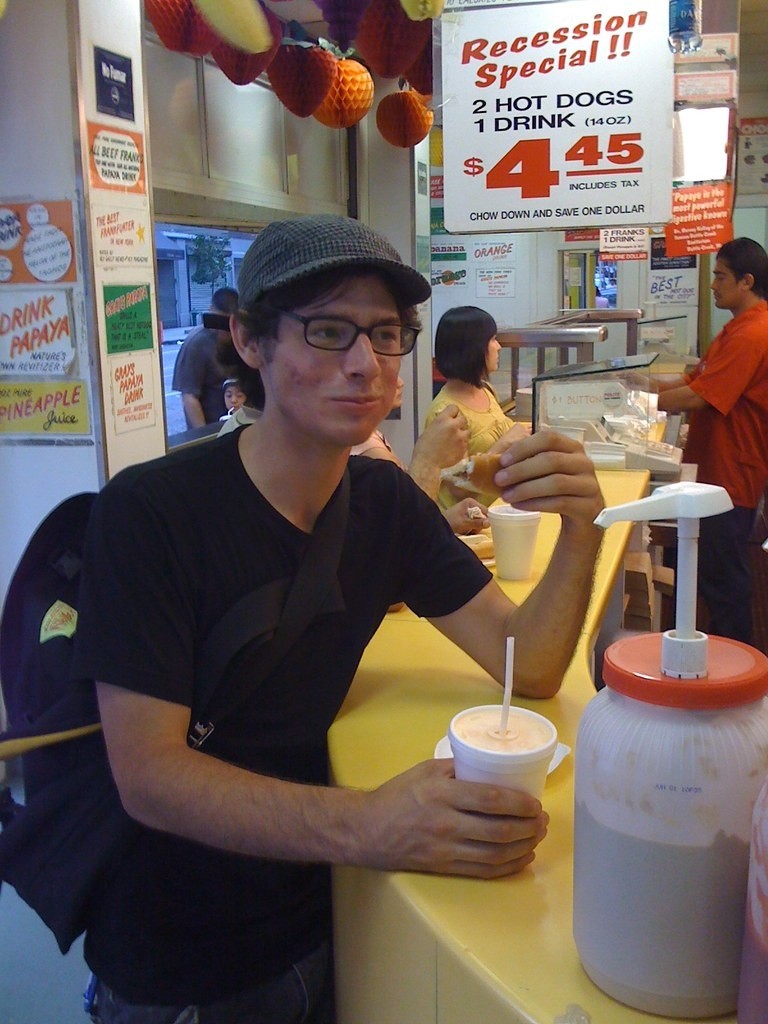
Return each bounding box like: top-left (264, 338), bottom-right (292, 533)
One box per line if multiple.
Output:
top-left (237, 219), bottom-right (431, 309)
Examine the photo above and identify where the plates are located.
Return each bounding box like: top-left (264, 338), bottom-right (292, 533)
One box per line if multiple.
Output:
top-left (433, 734), bottom-right (572, 777)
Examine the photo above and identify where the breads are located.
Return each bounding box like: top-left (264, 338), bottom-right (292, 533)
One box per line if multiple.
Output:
top-left (464, 452), bottom-right (507, 496)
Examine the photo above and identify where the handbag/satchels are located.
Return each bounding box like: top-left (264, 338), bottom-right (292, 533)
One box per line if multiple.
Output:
top-left (0, 452), bottom-right (353, 955)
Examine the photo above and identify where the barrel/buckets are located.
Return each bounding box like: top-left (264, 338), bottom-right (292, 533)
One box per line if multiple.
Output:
top-left (515, 388), bottom-right (532, 421)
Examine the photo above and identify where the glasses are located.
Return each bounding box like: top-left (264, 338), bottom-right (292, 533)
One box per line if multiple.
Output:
top-left (272, 297), bottom-right (421, 357)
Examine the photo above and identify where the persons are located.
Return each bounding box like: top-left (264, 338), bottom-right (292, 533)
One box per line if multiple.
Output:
top-left (617, 236), bottom-right (768, 646)
top-left (171, 286), bottom-right (239, 429)
top-left (222, 374), bottom-right (247, 413)
top-left (215, 305), bottom-right (532, 613)
top-left (73, 214), bottom-right (606, 1024)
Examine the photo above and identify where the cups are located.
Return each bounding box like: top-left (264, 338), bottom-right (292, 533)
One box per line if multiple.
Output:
top-left (447, 705), bottom-right (557, 801)
top-left (550, 425), bottom-right (585, 445)
top-left (219, 415), bottom-right (233, 421)
top-left (487, 505), bottom-right (539, 581)
